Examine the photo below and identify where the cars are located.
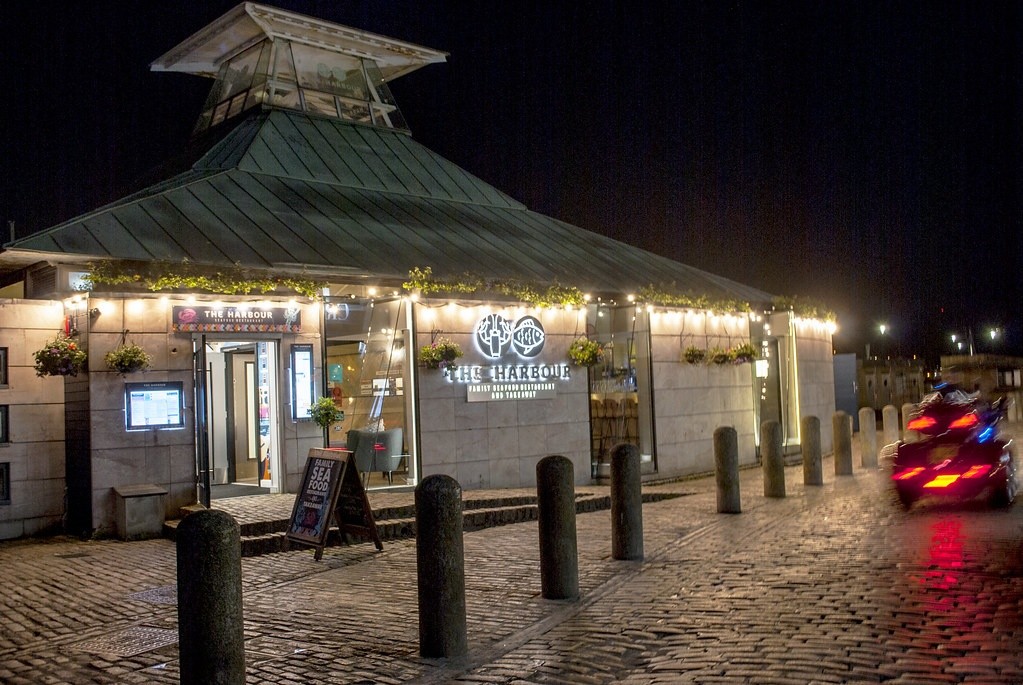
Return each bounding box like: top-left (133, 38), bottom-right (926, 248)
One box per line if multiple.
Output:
top-left (884, 382), bottom-right (1022, 509)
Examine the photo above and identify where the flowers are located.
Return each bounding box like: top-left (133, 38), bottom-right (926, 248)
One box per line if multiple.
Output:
top-left (417, 336), bottom-right (465, 378)
top-left (30, 329), bottom-right (88, 380)
top-left (567, 338), bottom-right (607, 369)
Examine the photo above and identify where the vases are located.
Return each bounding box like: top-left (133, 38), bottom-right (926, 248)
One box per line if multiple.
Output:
top-left (116, 360), bottom-right (141, 373)
top-left (581, 354), bottom-right (597, 368)
top-left (431, 350), bottom-right (456, 368)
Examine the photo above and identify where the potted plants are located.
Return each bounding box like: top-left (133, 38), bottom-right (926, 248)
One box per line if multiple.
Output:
top-left (680, 343), bottom-right (758, 369)
top-left (39, 356), bottom-right (72, 376)
top-left (104, 341), bottom-right (152, 380)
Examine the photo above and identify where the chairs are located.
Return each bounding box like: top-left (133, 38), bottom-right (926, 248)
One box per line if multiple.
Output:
top-left (345, 426), bottom-right (404, 486)
